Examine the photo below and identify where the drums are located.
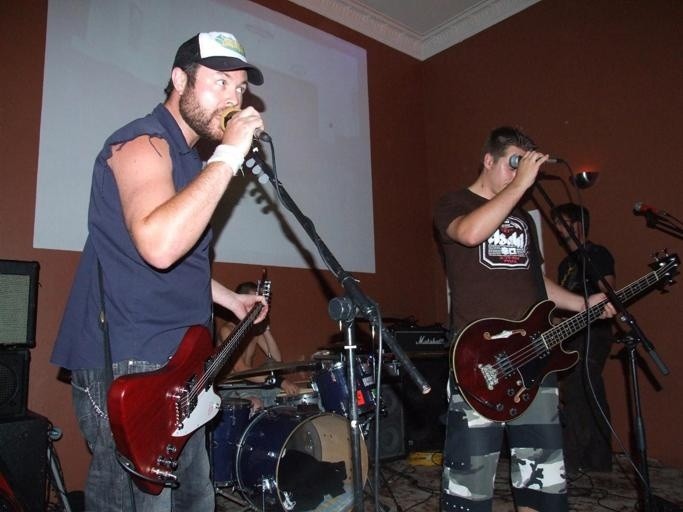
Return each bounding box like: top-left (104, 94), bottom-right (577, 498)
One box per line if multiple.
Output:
top-left (311, 360), bottom-right (376, 421)
top-left (235, 403), bottom-right (370, 512)
top-left (206, 396), bottom-right (253, 488)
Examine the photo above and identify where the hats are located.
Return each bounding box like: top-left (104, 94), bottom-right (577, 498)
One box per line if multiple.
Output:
top-left (174, 31), bottom-right (264, 85)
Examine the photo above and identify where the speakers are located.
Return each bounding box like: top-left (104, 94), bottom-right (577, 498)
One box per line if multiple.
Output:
top-left (0, 346), bottom-right (31, 417)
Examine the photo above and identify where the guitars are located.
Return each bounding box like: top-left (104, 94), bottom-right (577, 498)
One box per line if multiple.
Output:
top-left (107, 267), bottom-right (274, 497)
top-left (452, 249), bottom-right (682, 422)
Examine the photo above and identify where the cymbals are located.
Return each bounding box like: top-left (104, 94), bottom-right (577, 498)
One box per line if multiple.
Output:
top-left (226, 359), bottom-right (311, 380)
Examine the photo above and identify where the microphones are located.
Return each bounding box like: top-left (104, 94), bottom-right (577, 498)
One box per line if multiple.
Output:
top-left (508, 153), bottom-right (559, 168)
top-left (220, 108), bottom-right (273, 141)
top-left (634, 201), bottom-right (667, 217)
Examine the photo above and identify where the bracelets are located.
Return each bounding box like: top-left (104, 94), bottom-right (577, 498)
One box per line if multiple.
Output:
top-left (206, 143), bottom-right (246, 178)
top-left (266, 373), bottom-right (285, 389)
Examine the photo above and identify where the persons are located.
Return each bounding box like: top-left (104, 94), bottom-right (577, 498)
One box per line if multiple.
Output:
top-left (430, 125), bottom-right (620, 511)
top-left (47, 30), bottom-right (265, 511)
top-left (216, 279), bottom-right (298, 419)
top-left (553, 202), bottom-right (617, 474)
